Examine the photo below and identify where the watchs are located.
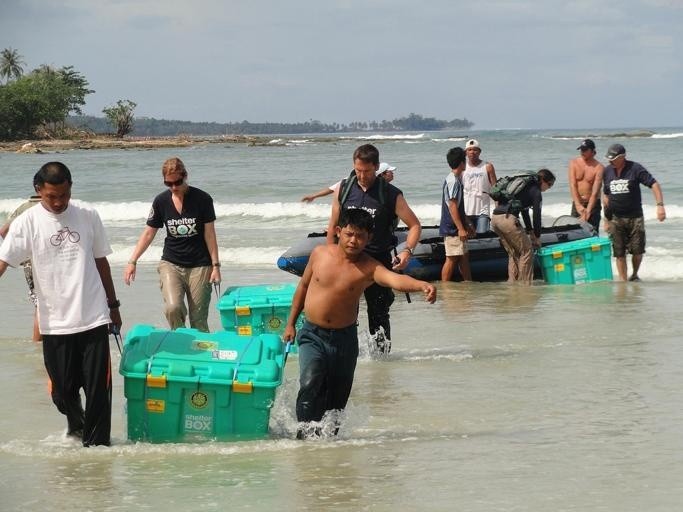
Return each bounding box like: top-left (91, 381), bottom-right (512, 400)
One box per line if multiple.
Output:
top-left (403, 246), bottom-right (415, 255)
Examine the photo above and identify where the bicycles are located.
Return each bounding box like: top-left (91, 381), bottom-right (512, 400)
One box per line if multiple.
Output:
top-left (51, 226), bottom-right (81, 248)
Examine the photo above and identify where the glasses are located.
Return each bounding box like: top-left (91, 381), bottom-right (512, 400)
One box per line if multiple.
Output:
top-left (164, 176), bottom-right (184, 187)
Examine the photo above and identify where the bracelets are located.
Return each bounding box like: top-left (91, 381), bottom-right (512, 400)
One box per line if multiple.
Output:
top-left (656, 202), bottom-right (664, 207)
top-left (129, 260), bottom-right (136, 265)
top-left (211, 263), bottom-right (221, 267)
top-left (105, 299), bottom-right (121, 308)
top-left (465, 223), bottom-right (474, 228)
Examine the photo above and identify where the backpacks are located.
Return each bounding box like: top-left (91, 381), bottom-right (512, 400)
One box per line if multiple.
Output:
top-left (489, 173), bottom-right (542, 211)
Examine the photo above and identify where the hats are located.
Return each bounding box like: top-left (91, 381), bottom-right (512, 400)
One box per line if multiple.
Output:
top-left (376, 163), bottom-right (397, 177)
top-left (576, 139), bottom-right (595, 150)
top-left (464, 138), bottom-right (482, 152)
top-left (604, 144), bottom-right (626, 161)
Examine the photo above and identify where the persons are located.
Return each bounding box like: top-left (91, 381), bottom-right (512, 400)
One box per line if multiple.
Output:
top-left (461, 139), bottom-right (497, 237)
top-left (602, 144), bottom-right (666, 281)
top-left (569, 139), bottom-right (604, 236)
top-left (302, 163), bottom-right (357, 204)
top-left (374, 163), bottom-right (397, 183)
top-left (285, 208), bottom-right (436, 438)
top-left (440, 147), bottom-right (477, 284)
top-left (0, 163), bottom-right (122, 447)
top-left (0, 171), bottom-right (44, 343)
top-left (325, 144), bottom-right (422, 356)
top-left (124, 158), bottom-right (221, 335)
top-left (491, 169), bottom-right (556, 287)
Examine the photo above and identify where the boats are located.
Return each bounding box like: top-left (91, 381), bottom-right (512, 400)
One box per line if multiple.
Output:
top-left (275, 214), bottom-right (599, 285)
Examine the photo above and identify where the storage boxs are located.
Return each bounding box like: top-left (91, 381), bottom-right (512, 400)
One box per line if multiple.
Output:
top-left (119, 323), bottom-right (285, 444)
top-left (535, 236), bottom-right (613, 285)
top-left (215, 283), bottom-right (303, 355)
